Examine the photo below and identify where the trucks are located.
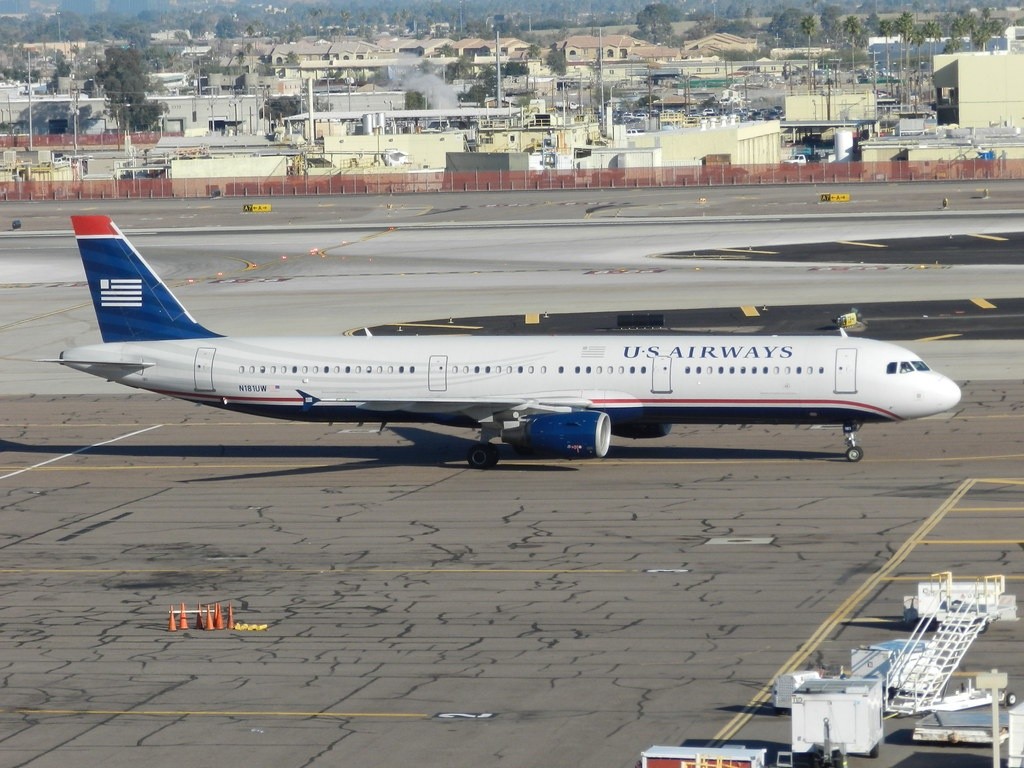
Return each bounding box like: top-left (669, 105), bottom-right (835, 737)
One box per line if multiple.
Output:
top-left (555, 102), bottom-right (584, 110)
top-left (781, 154), bottom-right (821, 164)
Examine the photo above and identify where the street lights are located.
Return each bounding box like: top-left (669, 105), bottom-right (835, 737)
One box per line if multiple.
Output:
top-left (609, 86), bottom-right (615, 106)
top-left (116, 104), bottom-right (130, 151)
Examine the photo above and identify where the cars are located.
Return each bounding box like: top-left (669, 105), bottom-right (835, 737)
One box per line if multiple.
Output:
top-left (627, 129), bottom-right (643, 133)
top-left (734, 105), bottom-right (783, 121)
top-left (702, 109), bottom-right (715, 116)
top-left (651, 110), bottom-right (659, 117)
top-left (623, 110), bottom-right (658, 121)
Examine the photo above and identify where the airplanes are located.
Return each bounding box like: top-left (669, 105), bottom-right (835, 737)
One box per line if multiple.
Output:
top-left (0, 214), bottom-right (961, 469)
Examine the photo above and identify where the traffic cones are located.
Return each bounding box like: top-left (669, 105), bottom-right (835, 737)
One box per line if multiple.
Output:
top-left (227, 603), bottom-right (235, 629)
top-left (167, 605), bottom-right (177, 631)
top-left (214, 603), bottom-right (225, 630)
top-left (205, 605), bottom-right (215, 630)
top-left (195, 603), bottom-right (204, 629)
top-left (180, 602), bottom-right (189, 629)
